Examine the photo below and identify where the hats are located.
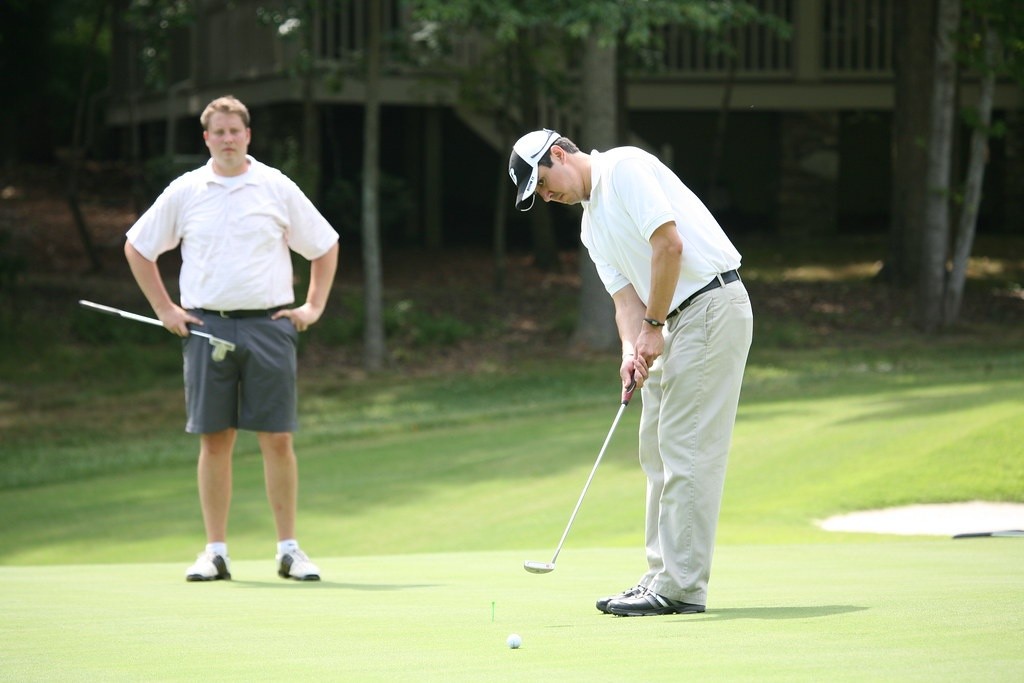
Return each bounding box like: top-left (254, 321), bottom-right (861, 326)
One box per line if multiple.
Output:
top-left (508, 128), bottom-right (561, 211)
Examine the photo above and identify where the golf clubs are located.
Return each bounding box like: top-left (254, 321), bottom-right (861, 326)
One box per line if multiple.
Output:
top-left (523, 361), bottom-right (648, 574)
top-left (77, 298), bottom-right (236, 363)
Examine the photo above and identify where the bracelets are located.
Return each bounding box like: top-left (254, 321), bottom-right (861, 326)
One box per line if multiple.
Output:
top-left (643, 319), bottom-right (665, 327)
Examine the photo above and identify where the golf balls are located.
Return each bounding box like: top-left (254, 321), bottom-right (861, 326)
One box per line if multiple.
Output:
top-left (507, 634), bottom-right (522, 649)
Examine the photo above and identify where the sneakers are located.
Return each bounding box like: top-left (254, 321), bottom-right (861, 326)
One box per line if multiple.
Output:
top-left (186, 551), bottom-right (231, 580)
top-left (596, 585), bottom-right (645, 613)
top-left (276, 549), bottom-right (320, 581)
top-left (606, 590), bottom-right (705, 616)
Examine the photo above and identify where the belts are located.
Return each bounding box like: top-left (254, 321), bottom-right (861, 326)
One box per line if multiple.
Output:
top-left (666, 270), bottom-right (738, 319)
top-left (195, 305), bottom-right (288, 319)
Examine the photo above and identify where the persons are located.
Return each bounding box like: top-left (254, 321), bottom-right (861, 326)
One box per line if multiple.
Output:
top-left (123, 94), bottom-right (341, 580)
top-left (509, 130), bottom-right (753, 615)
